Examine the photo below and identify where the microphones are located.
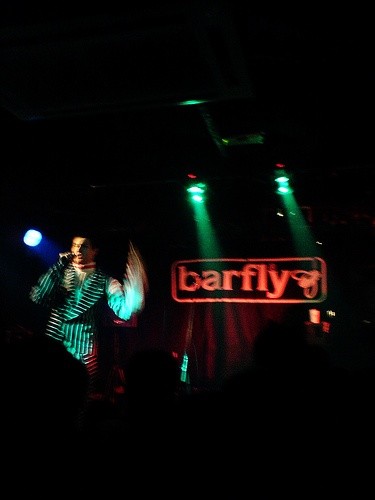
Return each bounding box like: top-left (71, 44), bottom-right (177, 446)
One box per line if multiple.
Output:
top-left (60, 253), bottom-right (76, 266)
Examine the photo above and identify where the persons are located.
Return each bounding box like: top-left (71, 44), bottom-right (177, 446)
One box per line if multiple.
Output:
top-left (27, 228), bottom-right (148, 407)
